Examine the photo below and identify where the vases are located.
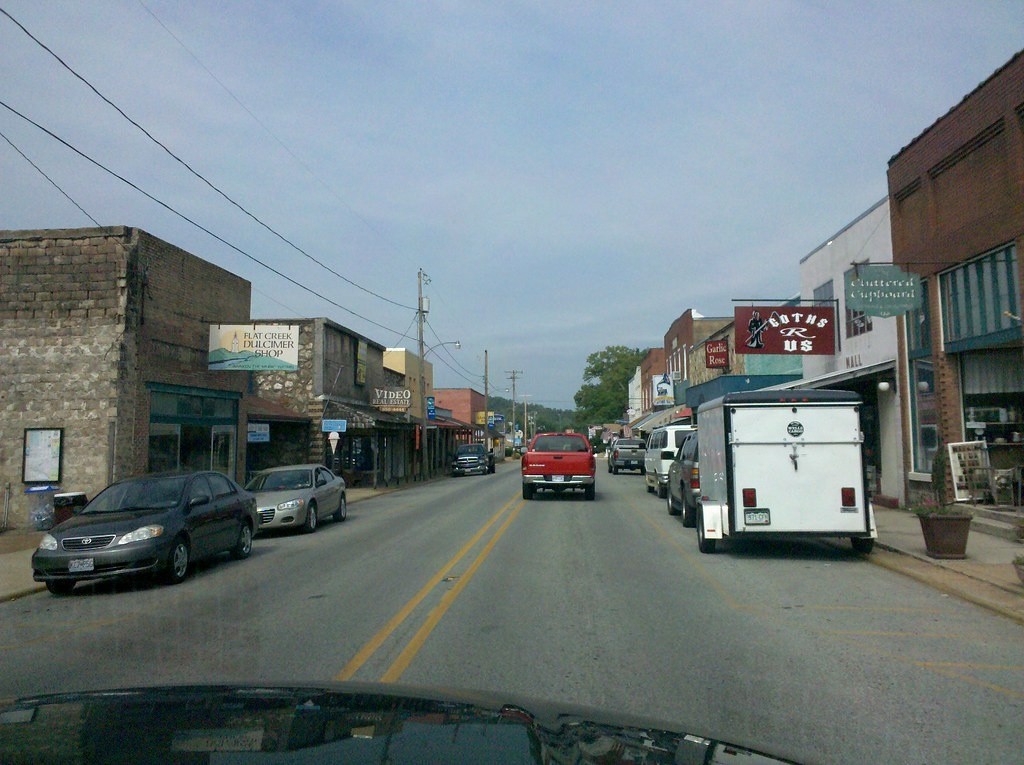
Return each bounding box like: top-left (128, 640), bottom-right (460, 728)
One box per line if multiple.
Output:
top-left (1013, 432), bottom-right (1020, 442)
top-left (915, 513), bottom-right (974, 559)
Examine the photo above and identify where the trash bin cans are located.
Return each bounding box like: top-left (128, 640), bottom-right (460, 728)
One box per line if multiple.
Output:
top-left (24, 486), bottom-right (59, 532)
top-left (54, 491), bottom-right (89, 527)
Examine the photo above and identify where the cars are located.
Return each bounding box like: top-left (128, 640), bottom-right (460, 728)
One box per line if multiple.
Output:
top-left (242, 465), bottom-right (347, 532)
top-left (31, 471), bottom-right (258, 596)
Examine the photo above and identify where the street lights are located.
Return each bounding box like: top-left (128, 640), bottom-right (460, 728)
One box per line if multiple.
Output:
top-left (422, 341), bottom-right (462, 481)
top-left (484, 389), bottom-right (509, 447)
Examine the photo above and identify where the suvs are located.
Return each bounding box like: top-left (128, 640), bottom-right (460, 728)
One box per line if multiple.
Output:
top-left (665, 429), bottom-right (699, 528)
top-left (865, 439), bottom-right (881, 470)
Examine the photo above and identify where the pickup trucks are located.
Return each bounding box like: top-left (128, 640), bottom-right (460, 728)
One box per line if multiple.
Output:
top-left (452, 444), bottom-right (496, 476)
top-left (521, 434), bottom-right (596, 501)
top-left (608, 440), bottom-right (647, 474)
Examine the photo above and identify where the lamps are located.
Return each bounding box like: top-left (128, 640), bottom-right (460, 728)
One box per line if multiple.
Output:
top-left (878, 375), bottom-right (896, 392)
top-left (918, 376), bottom-right (930, 392)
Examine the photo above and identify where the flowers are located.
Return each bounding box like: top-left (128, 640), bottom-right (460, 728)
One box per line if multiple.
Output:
top-left (901, 495), bottom-right (964, 519)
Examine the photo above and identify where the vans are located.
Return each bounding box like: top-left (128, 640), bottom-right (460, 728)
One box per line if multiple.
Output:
top-left (644, 425), bottom-right (698, 497)
top-left (921, 425), bottom-right (940, 470)
